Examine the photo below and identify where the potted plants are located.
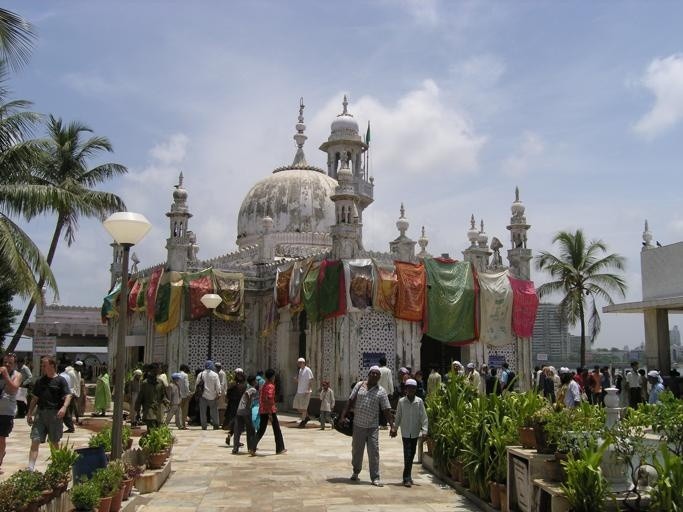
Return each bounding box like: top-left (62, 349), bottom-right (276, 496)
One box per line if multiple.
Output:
top-left (425, 357), bottom-right (604, 512)
top-left (0, 425), bottom-right (175, 512)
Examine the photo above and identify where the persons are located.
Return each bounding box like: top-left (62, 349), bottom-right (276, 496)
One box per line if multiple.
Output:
top-left (293, 358), bottom-right (313, 428)
top-left (320, 380), bottom-right (335, 429)
top-left (398, 367), bottom-right (426, 400)
top-left (91, 366), bottom-right (111, 417)
top-left (1, 350), bottom-right (22, 462)
top-left (391, 379), bottom-right (429, 487)
top-left (57, 360), bottom-right (85, 433)
top-left (14, 354), bottom-right (33, 419)
top-left (648, 368), bottom-right (682, 404)
top-left (379, 357), bottom-right (394, 430)
top-left (533, 361), bottom-right (648, 408)
top-left (223, 368), bottom-right (287, 455)
top-left (26, 355), bottom-right (72, 470)
top-left (127, 363), bottom-right (192, 430)
top-left (342, 366), bottom-right (396, 485)
top-left (193, 361), bottom-right (227, 429)
top-left (427, 360), bottom-right (516, 398)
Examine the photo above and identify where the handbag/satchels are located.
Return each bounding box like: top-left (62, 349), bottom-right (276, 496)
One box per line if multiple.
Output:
top-left (334, 418), bottom-right (353, 435)
top-left (195, 379), bottom-right (203, 397)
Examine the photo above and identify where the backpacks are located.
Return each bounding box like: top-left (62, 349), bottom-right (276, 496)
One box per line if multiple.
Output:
top-left (508, 371), bottom-right (515, 386)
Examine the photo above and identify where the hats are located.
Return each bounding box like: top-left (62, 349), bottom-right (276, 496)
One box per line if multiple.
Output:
top-left (366, 365), bottom-right (380, 376)
top-left (404, 378), bottom-right (418, 386)
top-left (297, 357), bottom-right (306, 362)
top-left (203, 360), bottom-right (223, 371)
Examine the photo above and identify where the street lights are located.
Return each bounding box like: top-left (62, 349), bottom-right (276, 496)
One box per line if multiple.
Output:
top-left (200, 294), bottom-right (222, 365)
top-left (100, 211), bottom-right (153, 465)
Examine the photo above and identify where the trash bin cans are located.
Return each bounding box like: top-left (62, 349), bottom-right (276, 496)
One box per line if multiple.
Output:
top-left (73, 447), bottom-right (107, 486)
